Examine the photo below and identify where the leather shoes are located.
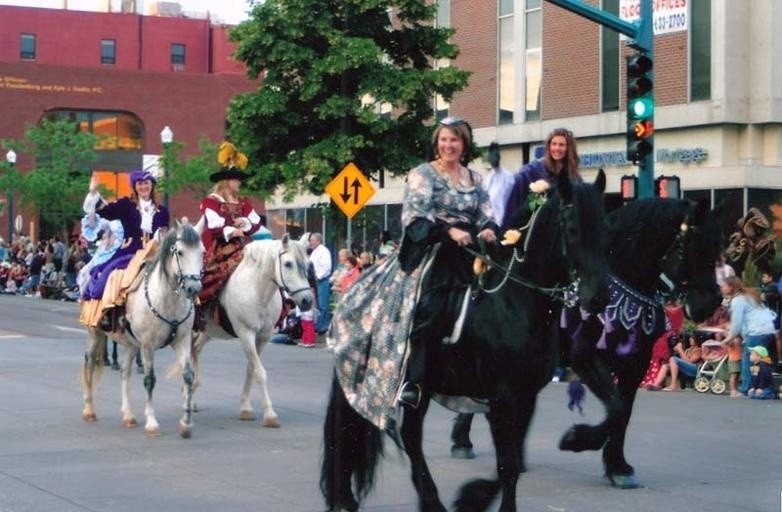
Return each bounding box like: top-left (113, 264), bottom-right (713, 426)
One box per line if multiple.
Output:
top-left (401, 389), bottom-right (418, 404)
top-left (101, 318), bottom-right (110, 325)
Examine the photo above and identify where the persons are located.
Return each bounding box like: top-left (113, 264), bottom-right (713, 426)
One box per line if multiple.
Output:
top-left (256, 215), bottom-right (401, 346)
top-left (194, 159), bottom-right (263, 331)
top-left (84, 169), bottom-right (172, 332)
top-left (397, 116), bottom-right (494, 408)
top-left (2, 227), bottom-right (106, 304)
top-left (75, 190), bottom-right (124, 303)
top-left (485, 126), bottom-right (584, 247)
top-left (628, 246), bottom-right (781, 403)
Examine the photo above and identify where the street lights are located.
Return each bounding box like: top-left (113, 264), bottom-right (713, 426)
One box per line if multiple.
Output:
top-left (160, 126), bottom-right (174, 210)
top-left (6, 150), bottom-right (18, 245)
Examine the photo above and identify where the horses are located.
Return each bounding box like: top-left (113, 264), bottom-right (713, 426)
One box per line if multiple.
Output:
top-left (80, 213), bottom-right (205, 439)
top-left (450, 194), bottom-right (736, 489)
top-left (164, 231), bottom-right (315, 428)
top-left (101, 334), bottom-right (145, 374)
top-left (319, 167), bottom-right (613, 512)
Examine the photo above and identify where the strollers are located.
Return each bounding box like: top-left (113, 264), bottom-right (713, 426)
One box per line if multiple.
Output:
top-left (693, 334), bottom-right (730, 394)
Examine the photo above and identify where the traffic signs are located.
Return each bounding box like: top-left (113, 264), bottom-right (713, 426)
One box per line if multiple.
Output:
top-left (326, 162), bottom-right (376, 219)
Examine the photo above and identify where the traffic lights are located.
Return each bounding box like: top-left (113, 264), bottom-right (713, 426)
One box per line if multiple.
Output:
top-left (627, 55), bottom-right (653, 160)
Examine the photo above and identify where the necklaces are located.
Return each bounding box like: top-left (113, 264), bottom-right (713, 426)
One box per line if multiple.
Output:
top-left (436, 159), bottom-right (463, 191)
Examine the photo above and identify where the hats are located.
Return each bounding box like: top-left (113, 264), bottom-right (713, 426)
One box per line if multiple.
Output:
top-left (130, 171), bottom-right (154, 180)
top-left (747, 345), bottom-right (769, 357)
top-left (210, 166), bottom-right (250, 182)
